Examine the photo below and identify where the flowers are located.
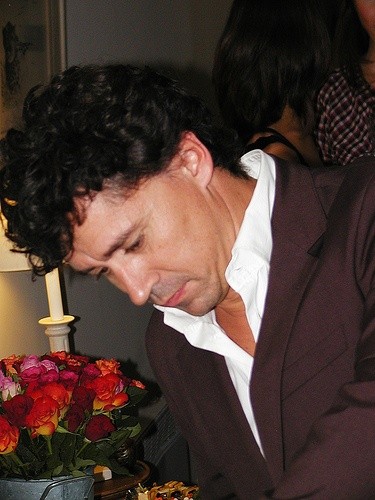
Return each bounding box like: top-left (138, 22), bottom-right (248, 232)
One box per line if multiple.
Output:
top-left (0, 350), bottom-right (146, 481)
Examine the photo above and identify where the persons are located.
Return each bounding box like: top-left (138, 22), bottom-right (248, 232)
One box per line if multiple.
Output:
top-left (212, 0), bottom-right (374, 169)
top-left (0, 64), bottom-right (374, 500)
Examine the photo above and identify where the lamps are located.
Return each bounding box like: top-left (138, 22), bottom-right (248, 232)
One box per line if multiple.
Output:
top-left (0, 200), bottom-right (75, 353)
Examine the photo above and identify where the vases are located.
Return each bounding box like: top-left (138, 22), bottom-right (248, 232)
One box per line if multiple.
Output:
top-left (0, 475), bottom-right (95, 500)
